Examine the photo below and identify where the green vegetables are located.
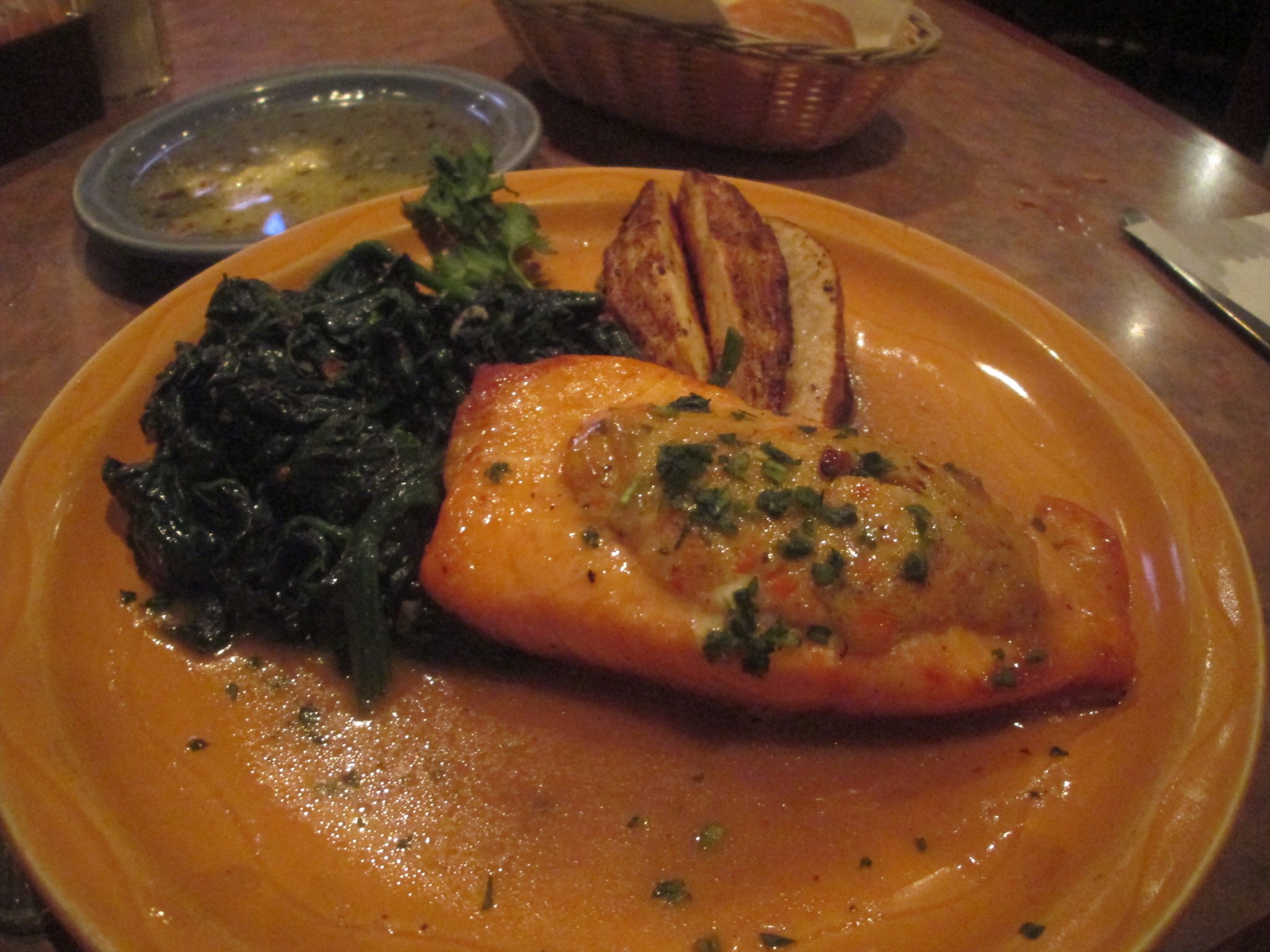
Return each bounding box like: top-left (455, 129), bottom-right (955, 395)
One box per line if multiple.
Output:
top-left (623, 327), bottom-right (930, 676)
top-left (102, 142), bottom-right (649, 714)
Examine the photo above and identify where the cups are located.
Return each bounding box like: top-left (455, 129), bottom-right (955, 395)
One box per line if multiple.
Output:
top-left (73, 0), bottom-right (175, 100)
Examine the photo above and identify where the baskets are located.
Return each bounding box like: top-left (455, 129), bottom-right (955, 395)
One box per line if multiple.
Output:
top-left (495, 0), bottom-right (941, 149)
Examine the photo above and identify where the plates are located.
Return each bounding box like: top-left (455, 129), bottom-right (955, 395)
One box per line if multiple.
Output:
top-left (0, 168), bottom-right (1267, 952)
top-left (72, 64), bottom-right (541, 261)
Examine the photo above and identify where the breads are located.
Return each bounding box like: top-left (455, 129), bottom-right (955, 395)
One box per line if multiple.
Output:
top-left (722, 0), bottom-right (858, 49)
top-left (602, 168), bottom-right (855, 426)
